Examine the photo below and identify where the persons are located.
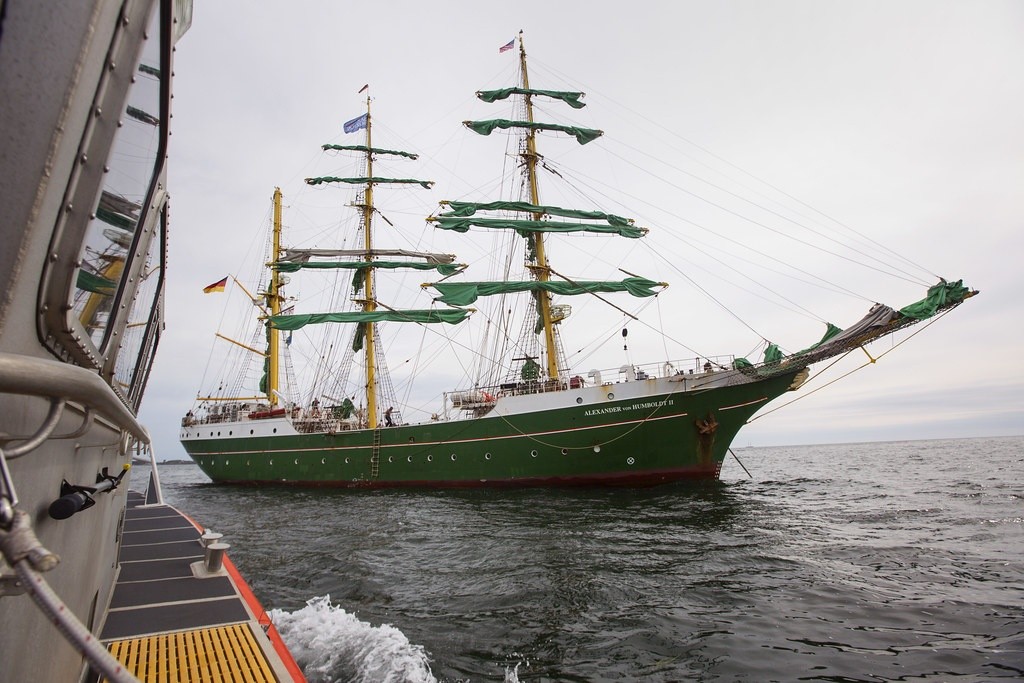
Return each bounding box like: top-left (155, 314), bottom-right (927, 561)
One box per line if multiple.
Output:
top-left (312, 398), bottom-right (320, 416)
top-left (186, 410), bottom-right (193, 426)
top-left (385, 407), bottom-right (393, 427)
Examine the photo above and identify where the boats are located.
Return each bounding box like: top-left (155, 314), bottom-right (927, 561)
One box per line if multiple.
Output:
top-left (0, 0), bottom-right (310, 683)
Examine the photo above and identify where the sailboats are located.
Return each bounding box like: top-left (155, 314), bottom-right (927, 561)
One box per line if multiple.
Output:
top-left (180, 28), bottom-right (979, 494)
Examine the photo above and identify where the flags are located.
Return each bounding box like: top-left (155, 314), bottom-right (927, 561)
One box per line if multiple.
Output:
top-left (499, 39), bottom-right (514, 53)
top-left (203, 275), bottom-right (228, 293)
top-left (344, 112), bottom-right (369, 134)
